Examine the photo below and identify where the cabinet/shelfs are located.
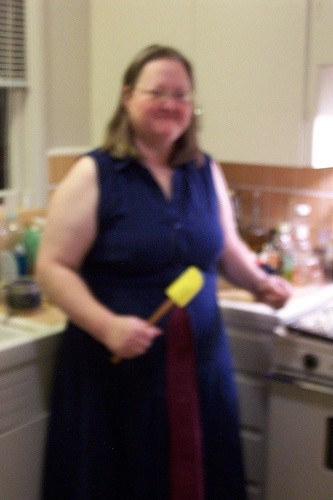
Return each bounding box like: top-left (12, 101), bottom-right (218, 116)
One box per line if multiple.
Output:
top-left (0, 326), bottom-right (296, 500)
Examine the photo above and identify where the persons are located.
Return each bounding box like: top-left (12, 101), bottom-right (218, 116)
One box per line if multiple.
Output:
top-left (34, 40), bottom-right (290, 499)
